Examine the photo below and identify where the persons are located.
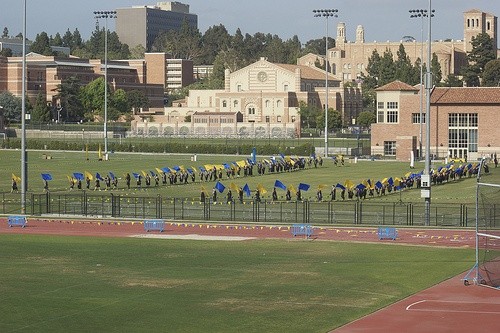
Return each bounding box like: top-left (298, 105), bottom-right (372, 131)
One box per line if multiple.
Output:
top-left (11, 148), bottom-right (497, 204)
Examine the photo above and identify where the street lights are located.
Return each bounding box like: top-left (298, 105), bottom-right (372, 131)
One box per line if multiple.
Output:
top-left (313, 8), bottom-right (339, 158)
top-left (92, 10), bottom-right (118, 124)
top-left (409, 9), bottom-right (435, 161)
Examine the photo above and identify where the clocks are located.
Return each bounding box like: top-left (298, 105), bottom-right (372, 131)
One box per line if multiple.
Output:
top-left (257, 71), bottom-right (268, 82)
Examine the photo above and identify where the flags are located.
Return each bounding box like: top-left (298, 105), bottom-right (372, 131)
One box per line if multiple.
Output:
top-left (298, 182), bottom-right (310, 190)
top-left (109, 172), bottom-right (115, 181)
top-left (243, 183), bottom-right (251, 197)
top-left (356, 183), bottom-right (366, 189)
top-left (274, 179), bottom-right (286, 190)
top-left (256, 182), bottom-right (267, 197)
top-left (96, 172), bottom-right (104, 181)
top-left (186, 168), bottom-right (193, 174)
top-left (236, 160), bottom-right (247, 168)
top-left (336, 183), bottom-right (346, 190)
top-left (215, 181), bottom-right (225, 193)
top-left (41, 173), bottom-right (52, 180)
top-left (73, 172), bottom-right (83, 180)
top-left (85, 171), bottom-right (93, 181)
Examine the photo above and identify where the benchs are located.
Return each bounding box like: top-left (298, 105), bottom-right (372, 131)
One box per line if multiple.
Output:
top-left (377, 226), bottom-right (399, 241)
top-left (290, 223), bottom-right (318, 240)
top-left (7, 214), bottom-right (29, 229)
top-left (143, 218), bottom-right (167, 233)
